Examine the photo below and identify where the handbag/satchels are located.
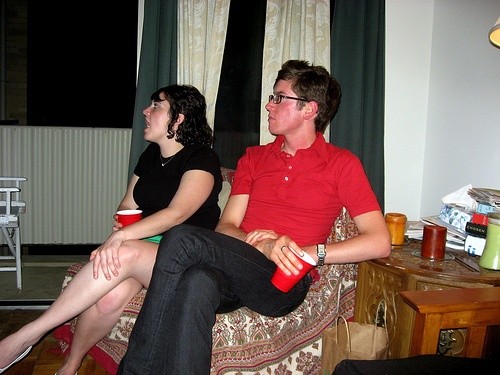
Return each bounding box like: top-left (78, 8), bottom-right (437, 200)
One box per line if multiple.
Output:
top-left (322, 299), bottom-right (389, 375)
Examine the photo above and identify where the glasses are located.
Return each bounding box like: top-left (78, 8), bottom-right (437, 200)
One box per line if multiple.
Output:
top-left (269, 95), bottom-right (308, 104)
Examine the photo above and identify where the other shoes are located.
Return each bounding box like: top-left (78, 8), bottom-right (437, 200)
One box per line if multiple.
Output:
top-left (54, 370), bottom-right (78, 375)
top-left (0, 345), bottom-right (32, 374)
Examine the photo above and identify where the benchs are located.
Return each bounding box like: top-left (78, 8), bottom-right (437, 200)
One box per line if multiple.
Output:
top-left (53, 165), bottom-right (347, 373)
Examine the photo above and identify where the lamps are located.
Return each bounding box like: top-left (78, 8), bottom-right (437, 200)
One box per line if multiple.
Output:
top-left (489, 16), bottom-right (500, 49)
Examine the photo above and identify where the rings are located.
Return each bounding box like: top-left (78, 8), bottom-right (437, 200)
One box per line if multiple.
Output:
top-left (280, 245), bottom-right (287, 251)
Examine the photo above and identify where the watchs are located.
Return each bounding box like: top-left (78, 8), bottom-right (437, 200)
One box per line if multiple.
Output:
top-left (316, 242), bottom-right (327, 266)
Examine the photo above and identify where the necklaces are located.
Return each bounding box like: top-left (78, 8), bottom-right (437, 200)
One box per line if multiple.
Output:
top-left (159, 152), bottom-right (177, 167)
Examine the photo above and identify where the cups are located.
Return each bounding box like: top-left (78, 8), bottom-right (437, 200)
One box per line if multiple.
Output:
top-left (117, 210), bottom-right (143, 228)
top-left (271, 246), bottom-right (316, 292)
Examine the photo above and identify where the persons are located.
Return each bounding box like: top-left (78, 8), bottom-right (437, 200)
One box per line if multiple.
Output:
top-left (117, 60), bottom-right (391, 375)
top-left (0, 85), bottom-right (224, 375)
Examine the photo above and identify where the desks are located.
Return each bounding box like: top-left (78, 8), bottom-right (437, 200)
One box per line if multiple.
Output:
top-left (353, 241), bottom-right (500, 360)
